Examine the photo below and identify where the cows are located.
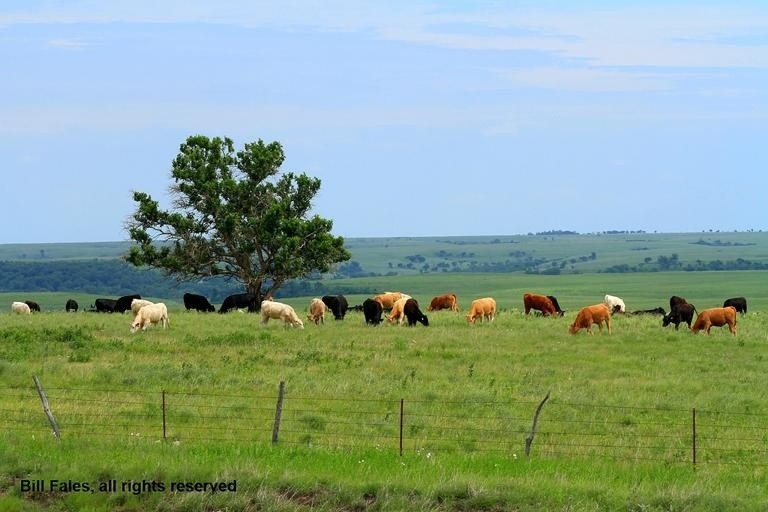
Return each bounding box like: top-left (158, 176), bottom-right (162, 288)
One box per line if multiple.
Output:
top-left (306, 294), bottom-right (348, 326)
top-left (568, 303), bottom-right (612, 337)
top-left (426, 294), bottom-right (458, 312)
top-left (362, 291), bottom-right (429, 327)
top-left (91, 294), bottom-right (156, 317)
top-left (128, 302), bottom-right (172, 334)
top-left (723, 297), bottom-right (747, 318)
top-left (689, 306), bottom-right (737, 337)
top-left (523, 292), bottom-right (566, 317)
top-left (65, 299), bottom-right (78, 313)
top-left (466, 297), bottom-right (496, 324)
top-left (217, 293), bottom-right (258, 314)
top-left (260, 300), bottom-right (304, 329)
top-left (183, 293), bottom-right (215, 313)
top-left (602, 294), bottom-right (698, 331)
top-left (11, 300), bottom-right (41, 315)
